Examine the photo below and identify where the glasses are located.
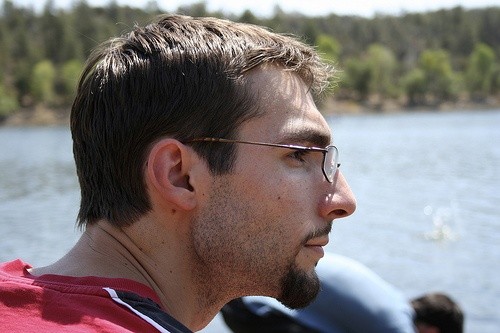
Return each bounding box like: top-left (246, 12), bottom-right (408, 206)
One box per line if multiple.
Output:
top-left (184, 134), bottom-right (341, 183)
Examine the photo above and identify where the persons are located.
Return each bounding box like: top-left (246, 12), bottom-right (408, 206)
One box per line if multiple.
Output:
top-left (0, 14), bottom-right (358, 333)
top-left (411, 293), bottom-right (463, 333)
